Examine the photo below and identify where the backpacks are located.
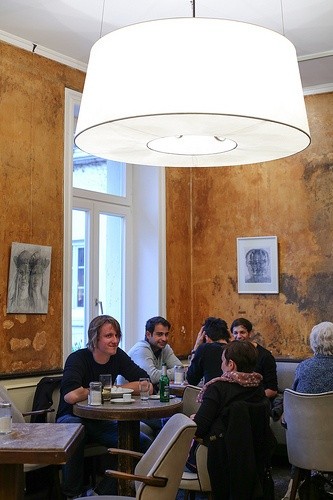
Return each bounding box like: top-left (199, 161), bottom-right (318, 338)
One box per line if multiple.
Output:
top-left (96, 478), bottom-right (119, 495)
top-left (298, 475), bottom-right (333, 500)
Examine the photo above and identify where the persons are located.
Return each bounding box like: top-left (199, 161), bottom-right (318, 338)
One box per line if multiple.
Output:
top-left (120, 309), bottom-right (277, 500)
top-left (0, 382), bottom-right (25, 424)
top-left (56, 315), bottom-right (152, 500)
top-left (185, 340), bottom-right (272, 500)
top-left (129, 316), bottom-right (184, 438)
top-left (281, 322), bottom-right (333, 500)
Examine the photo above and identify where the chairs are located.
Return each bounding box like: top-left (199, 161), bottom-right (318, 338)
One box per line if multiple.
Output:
top-left (0, 375), bottom-right (278, 500)
top-left (280, 388), bottom-right (333, 500)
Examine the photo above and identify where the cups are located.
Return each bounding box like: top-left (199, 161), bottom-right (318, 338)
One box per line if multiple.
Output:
top-left (139, 378), bottom-right (150, 400)
top-left (99, 374), bottom-right (112, 401)
top-left (123, 393), bottom-right (131, 401)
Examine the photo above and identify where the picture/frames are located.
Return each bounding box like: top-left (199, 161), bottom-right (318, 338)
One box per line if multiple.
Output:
top-left (236, 236), bottom-right (280, 294)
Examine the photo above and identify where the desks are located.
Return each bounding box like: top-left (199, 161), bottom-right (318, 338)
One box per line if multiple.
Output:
top-left (73, 393), bottom-right (184, 497)
top-left (167, 381), bottom-right (204, 396)
top-left (0, 422), bottom-right (87, 500)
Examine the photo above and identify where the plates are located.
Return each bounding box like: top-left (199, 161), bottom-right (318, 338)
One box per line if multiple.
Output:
top-left (110, 388), bottom-right (134, 397)
top-left (150, 394), bottom-right (176, 399)
top-left (110, 398), bottom-right (135, 403)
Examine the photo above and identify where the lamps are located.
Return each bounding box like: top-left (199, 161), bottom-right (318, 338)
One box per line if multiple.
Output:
top-left (74, 1), bottom-right (312, 167)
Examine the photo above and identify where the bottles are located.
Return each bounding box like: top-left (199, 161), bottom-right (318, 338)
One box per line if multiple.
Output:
top-left (0, 403), bottom-right (13, 434)
top-left (173, 366), bottom-right (184, 384)
top-left (89, 381), bottom-right (102, 405)
top-left (159, 363), bottom-right (169, 401)
